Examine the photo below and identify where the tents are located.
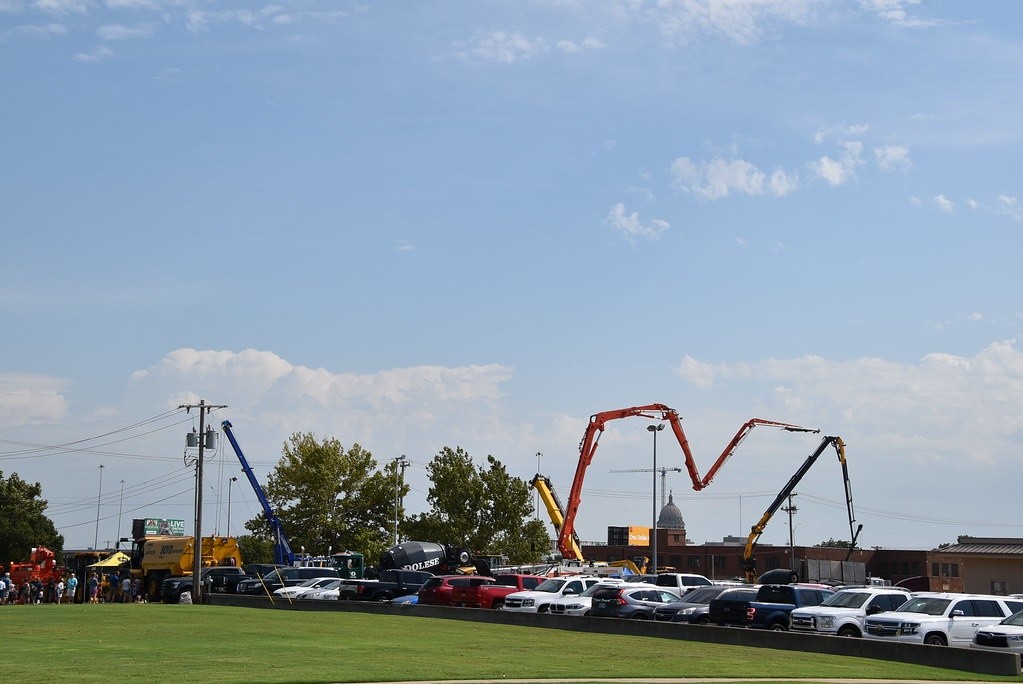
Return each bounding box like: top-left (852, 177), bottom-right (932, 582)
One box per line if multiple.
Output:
top-left (84, 551), bottom-right (131, 602)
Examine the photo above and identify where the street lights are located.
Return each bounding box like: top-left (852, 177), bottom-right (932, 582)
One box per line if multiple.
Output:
top-left (535, 451), bottom-right (544, 520)
top-left (647, 423), bottom-right (668, 574)
top-left (116, 480), bottom-right (125, 542)
top-left (227, 476), bottom-right (238, 538)
top-left (94, 463), bottom-right (105, 551)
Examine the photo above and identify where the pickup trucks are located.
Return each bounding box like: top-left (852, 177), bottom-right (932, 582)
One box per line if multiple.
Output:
top-left (708, 583), bottom-right (835, 632)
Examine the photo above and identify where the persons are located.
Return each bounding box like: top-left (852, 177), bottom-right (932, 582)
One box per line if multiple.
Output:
top-left (47, 576), bottom-right (56, 604)
top-left (57, 578), bottom-right (64, 605)
top-left (67, 573), bottom-right (78, 604)
top-left (18, 576), bottom-right (42, 605)
top-left (122, 575), bottom-right (142, 603)
top-left (88, 572), bottom-right (102, 604)
top-left (110, 571), bottom-right (121, 603)
top-left (0, 572), bottom-right (15, 605)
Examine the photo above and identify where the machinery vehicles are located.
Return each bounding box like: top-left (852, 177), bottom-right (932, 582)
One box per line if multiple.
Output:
top-left (7, 545), bottom-right (72, 603)
top-left (556, 403), bottom-right (821, 581)
top-left (528, 472), bottom-right (678, 577)
top-left (129, 534), bottom-right (244, 602)
top-left (75, 551), bottom-right (111, 605)
top-left (219, 419), bottom-right (336, 567)
top-left (329, 540), bottom-right (449, 582)
top-left (741, 434), bottom-right (864, 586)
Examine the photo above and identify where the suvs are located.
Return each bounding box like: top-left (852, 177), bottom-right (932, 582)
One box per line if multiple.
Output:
top-left (236, 565), bottom-right (342, 596)
top-left (788, 588), bottom-right (927, 637)
top-left (861, 591), bottom-right (1023, 648)
top-left (969, 608), bottom-right (1023, 670)
top-left (160, 566), bottom-right (246, 605)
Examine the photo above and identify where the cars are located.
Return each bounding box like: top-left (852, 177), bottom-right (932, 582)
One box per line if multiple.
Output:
top-left (275, 562), bottom-right (801, 626)
